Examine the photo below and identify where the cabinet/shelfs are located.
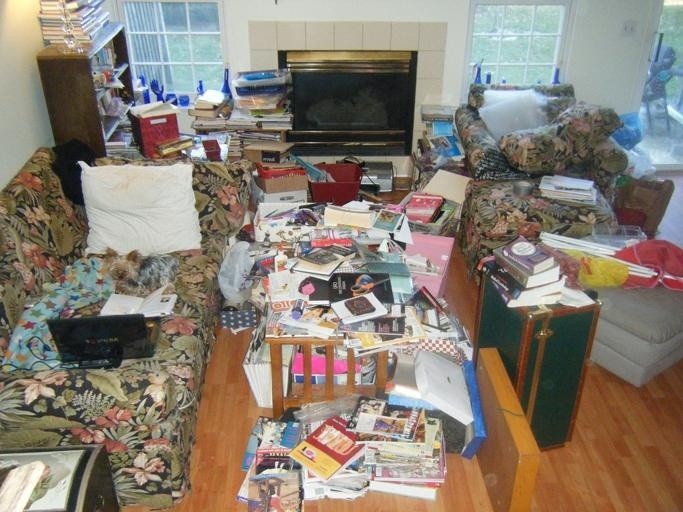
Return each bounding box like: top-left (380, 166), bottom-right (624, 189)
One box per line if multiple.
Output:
top-left (37, 23), bottom-right (139, 155)
top-left (0, 442), bottom-right (123, 511)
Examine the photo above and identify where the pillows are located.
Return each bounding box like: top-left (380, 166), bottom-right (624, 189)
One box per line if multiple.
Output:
top-left (75, 160), bottom-right (201, 257)
top-left (548, 99), bottom-right (624, 168)
top-left (498, 125), bottom-right (568, 175)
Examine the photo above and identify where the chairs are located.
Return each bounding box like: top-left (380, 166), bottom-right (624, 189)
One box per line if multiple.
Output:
top-left (267, 335), bottom-right (387, 417)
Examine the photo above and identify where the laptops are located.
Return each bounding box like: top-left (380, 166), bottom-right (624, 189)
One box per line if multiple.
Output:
top-left (45, 312), bottom-right (162, 361)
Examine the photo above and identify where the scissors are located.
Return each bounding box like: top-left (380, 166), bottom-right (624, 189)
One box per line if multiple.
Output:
top-left (351, 279), bottom-right (390, 297)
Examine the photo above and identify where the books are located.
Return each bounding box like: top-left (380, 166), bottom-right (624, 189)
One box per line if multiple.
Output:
top-left (97, 87), bottom-right (122, 122)
top-left (154, 137), bottom-right (194, 158)
top-left (475, 234), bottom-right (566, 308)
top-left (194, 130), bottom-right (280, 162)
top-left (90, 39), bottom-right (118, 77)
top-left (234, 396), bottom-right (447, 511)
top-left (538, 174), bottom-right (596, 208)
top-left (0, 460), bottom-right (43, 511)
top-left (249, 191), bottom-right (472, 356)
top-left (36, 0), bottom-right (112, 49)
top-left (104, 127), bottom-right (141, 155)
top-left (423, 120), bottom-right (465, 161)
top-left (128, 99), bottom-right (180, 119)
top-left (188, 91), bottom-right (292, 130)
top-left (97, 284), bottom-right (177, 318)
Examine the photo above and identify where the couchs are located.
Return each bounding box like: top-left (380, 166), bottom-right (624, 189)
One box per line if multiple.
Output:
top-left (455, 83), bottom-right (629, 205)
top-left (0, 147), bottom-right (252, 511)
top-left (459, 177), bottom-right (620, 281)
top-left (572, 233), bottom-right (683, 388)
top-left (476, 89), bottom-right (544, 142)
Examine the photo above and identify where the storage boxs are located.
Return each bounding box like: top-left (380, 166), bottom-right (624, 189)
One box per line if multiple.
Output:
top-left (250, 161), bottom-right (308, 202)
top-left (591, 224), bottom-right (647, 248)
top-left (126, 111), bottom-right (181, 158)
top-left (398, 233), bottom-right (457, 300)
top-left (307, 164), bottom-right (362, 202)
top-left (218, 307), bottom-right (259, 330)
top-left (241, 329), bottom-right (290, 410)
top-left (397, 188), bottom-right (459, 236)
top-left (475, 268), bottom-right (602, 453)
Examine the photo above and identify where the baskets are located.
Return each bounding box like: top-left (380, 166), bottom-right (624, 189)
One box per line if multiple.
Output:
top-left (217, 297), bottom-right (259, 329)
top-left (417, 335), bottom-right (465, 367)
top-left (255, 161), bottom-right (305, 179)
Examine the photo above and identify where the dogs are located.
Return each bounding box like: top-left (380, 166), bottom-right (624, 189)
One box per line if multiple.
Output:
top-left (103, 247), bottom-right (179, 297)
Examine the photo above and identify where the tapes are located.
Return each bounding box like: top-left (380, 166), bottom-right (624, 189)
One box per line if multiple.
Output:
top-left (512, 180), bottom-right (535, 195)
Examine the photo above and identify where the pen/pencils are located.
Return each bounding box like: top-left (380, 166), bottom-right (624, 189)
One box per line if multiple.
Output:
top-left (290, 261), bottom-right (299, 271)
top-left (264, 209), bottom-right (278, 218)
